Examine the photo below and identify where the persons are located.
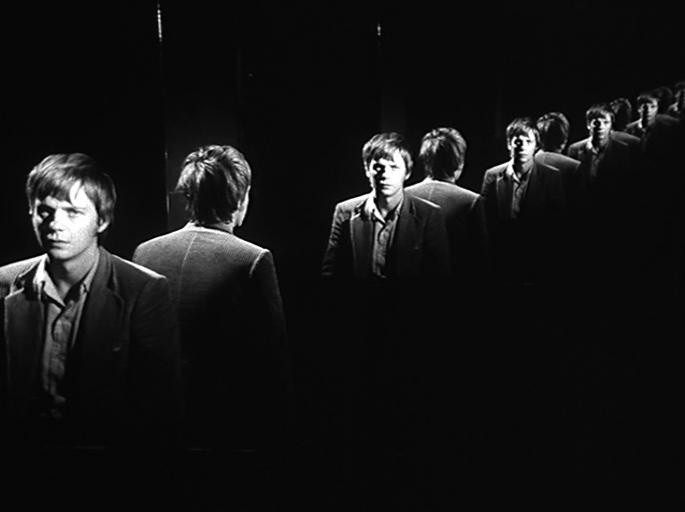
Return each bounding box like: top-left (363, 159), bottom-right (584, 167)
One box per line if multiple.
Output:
top-left (568, 103), bottom-right (631, 176)
top-left (532, 112), bottom-right (583, 177)
top-left (609, 98), bottom-right (646, 150)
top-left (667, 87), bottom-right (684, 119)
top-left (623, 95), bottom-right (672, 146)
top-left (321, 131), bottom-right (453, 309)
top-left (482, 117), bottom-right (566, 233)
top-left (0, 151), bottom-right (184, 462)
top-left (650, 88), bottom-right (678, 127)
top-left (133, 143), bottom-right (288, 371)
top-left (404, 127), bottom-right (488, 259)
